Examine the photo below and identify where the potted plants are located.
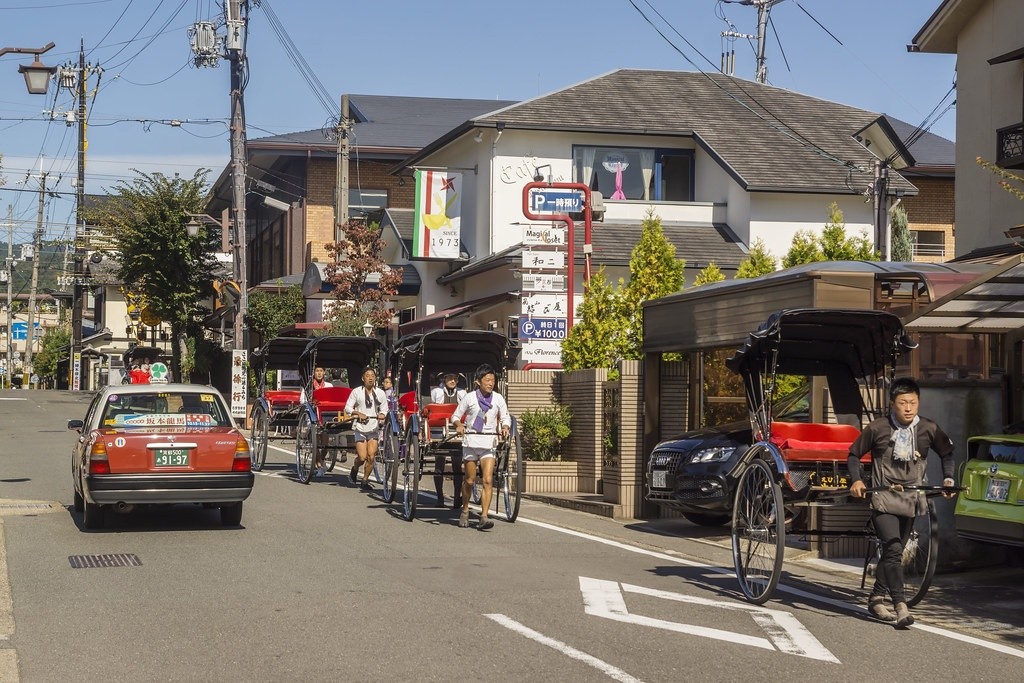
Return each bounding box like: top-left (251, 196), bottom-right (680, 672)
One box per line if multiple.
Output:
top-left (511, 404), bottom-right (580, 492)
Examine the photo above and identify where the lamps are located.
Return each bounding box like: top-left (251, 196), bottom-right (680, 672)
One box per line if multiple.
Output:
top-left (473, 131), bottom-right (484, 143)
top-left (398, 173), bottom-right (405, 186)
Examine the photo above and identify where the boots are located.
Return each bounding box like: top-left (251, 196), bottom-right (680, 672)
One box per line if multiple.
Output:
top-left (893, 601), bottom-right (914, 629)
top-left (868, 596), bottom-right (896, 621)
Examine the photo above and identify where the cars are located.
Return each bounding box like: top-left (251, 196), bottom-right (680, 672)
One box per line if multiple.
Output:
top-left (954, 419), bottom-right (1024, 550)
top-left (645, 380), bottom-right (886, 529)
top-left (68, 383), bottom-right (255, 527)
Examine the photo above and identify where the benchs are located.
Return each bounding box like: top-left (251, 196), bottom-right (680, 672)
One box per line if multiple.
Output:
top-left (421, 404), bottom-right (466, 426)
top-left (756, 422), bottom-right (872, 461)
top-left (266, 390), bottom-right (301, 414)
top-left (313, 387), bottom-right (352, 424)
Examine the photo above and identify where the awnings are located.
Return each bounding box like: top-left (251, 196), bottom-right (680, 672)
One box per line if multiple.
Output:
top-left (280, 319), bottom-right (386, 329)
top-left (398, 289), bottom-right (521, 335)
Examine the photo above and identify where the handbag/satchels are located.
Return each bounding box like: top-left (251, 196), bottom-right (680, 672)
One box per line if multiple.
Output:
top-left (869, 472), bottom-right (919, 517)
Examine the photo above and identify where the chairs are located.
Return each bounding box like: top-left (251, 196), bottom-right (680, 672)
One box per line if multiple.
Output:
top-left (110, 409), bottom-right (134, 419)
top-left (178, 406), bottom-right (204, 414)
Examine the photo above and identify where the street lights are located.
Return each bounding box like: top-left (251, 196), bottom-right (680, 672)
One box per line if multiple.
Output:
top-left (180, 208), bottom-right (248, 351)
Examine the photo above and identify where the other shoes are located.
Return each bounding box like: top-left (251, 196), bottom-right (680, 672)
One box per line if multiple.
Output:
top-left (349, 466), bottom-right (358, 486)
top-left (341, 450), bottom-right (347, 462)
top-left (359, 481), bottom-right (373, 492)
top-left (477, 517), bottom-right (494, 530)
top-left (455, 496), bottom-right (463, 508)
top-left (459, 513), bottom-right (470, 527)
top-left (435, 497), bottom-right (444, 509)
top-left (316, 467), bottom-right (325, 477)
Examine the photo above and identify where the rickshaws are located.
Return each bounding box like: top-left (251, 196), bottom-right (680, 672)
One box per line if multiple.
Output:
top-left (119, 346), bottom-right (167, 384)
top-left (725, 306), bottom-right (970, 607)
top-left (246, 329), bottom-right (523, 522)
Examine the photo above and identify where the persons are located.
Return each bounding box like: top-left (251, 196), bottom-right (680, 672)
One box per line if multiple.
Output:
top-left (345, 368), bottom-right (388, 492)
top-left (451, 364), bottom-right (510, 528)
top-left (383, 376), bottom-right (395, 414)
top-left (847, 378), bottom-right (957, 627)
top-left (328, 369), bottom-right (347, 463)
top-left (140, 356), bottom-right (153, 373)
top-left (131, 357), bottom-right (141, 371)
top-left (299, 367), bottom-right (333, 470)
top-left (430, 356), bottom-right (467, 509)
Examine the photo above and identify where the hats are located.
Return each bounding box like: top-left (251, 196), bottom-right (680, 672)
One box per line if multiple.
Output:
top-left (437, 370), bottom-right (467, 389)
